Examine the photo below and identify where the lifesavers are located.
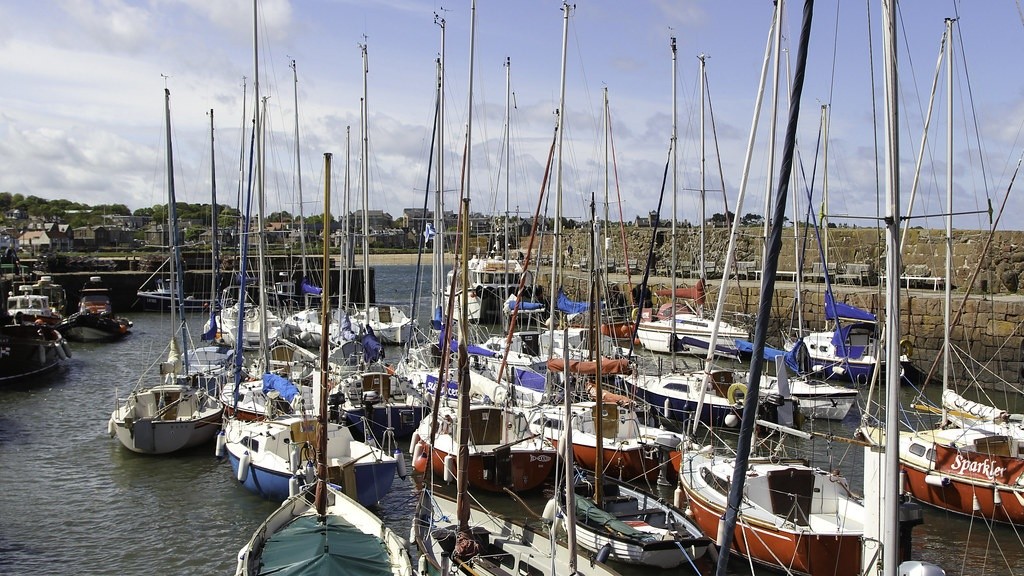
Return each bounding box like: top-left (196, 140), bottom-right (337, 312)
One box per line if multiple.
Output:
top-left (899, 339), bottom-right (913, 358)
top-left (727, 382), bottom-right (748, 404)
top-left (632, 307), bottom-right (639, 321)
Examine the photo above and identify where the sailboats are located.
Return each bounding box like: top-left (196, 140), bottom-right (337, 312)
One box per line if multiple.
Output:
top-left (0, 0), bottom-right (1024, 576)
